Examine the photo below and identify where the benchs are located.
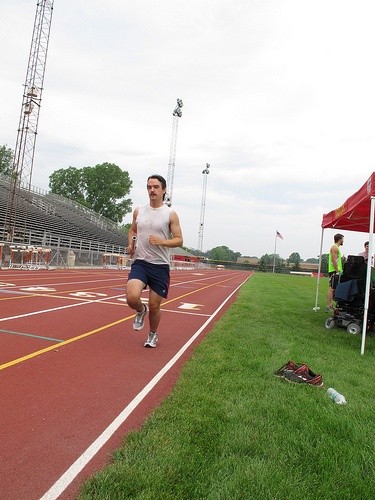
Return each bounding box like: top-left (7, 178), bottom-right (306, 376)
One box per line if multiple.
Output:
top-left (0, 181), bottom-right (129, 254)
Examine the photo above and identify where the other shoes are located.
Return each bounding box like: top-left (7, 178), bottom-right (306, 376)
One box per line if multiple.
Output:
top-left (325, 306), bottom-right (335, 312)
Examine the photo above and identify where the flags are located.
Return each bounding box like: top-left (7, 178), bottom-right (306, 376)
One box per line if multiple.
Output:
top-left (276, 231), bottom-right (283, 240)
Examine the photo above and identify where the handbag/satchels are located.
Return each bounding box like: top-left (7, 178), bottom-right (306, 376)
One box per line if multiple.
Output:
top-left (331, 271), bottom-right (340, 288)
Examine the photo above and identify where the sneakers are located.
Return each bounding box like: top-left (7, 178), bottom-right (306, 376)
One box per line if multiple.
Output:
top-left (133, 304), bottom-right (147, 330)
top-left (144, 332), bottom-right (158, 348)
top-left (272, 360), bottom-right (300, 376)
top-left (284, 365), bottom-right (323, 387)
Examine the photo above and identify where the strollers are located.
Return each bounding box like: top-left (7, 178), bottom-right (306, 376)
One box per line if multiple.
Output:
top-left (324, 255), bottom-right (375, 335)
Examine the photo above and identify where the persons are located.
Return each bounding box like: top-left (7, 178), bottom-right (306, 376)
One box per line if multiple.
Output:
top-left (126, 174), bottom-right (183, 348)
top-left (327, 233), bottom-right (344, 310)
top-left (359, 241), bottom-right (374, 265)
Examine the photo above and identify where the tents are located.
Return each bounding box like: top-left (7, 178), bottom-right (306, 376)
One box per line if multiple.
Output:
top-left (313, 172), bottom-right (375, 355)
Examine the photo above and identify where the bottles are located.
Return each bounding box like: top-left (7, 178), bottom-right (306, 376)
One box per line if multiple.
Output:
top-left (327, 387), bottom-right (346, 404)
